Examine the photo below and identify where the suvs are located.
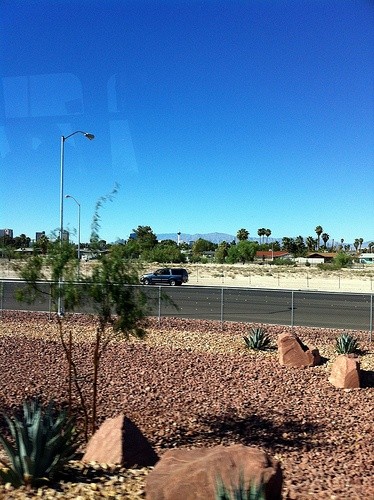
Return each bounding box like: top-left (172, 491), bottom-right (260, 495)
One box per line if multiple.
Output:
top-left (141, 266), bottom-right (189, 286)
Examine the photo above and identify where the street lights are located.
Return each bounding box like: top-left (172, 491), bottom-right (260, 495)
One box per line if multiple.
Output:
top-left (58, 130), bottom-right (95, 243)
top-left (64, 195), bottom-right (81, 278)
